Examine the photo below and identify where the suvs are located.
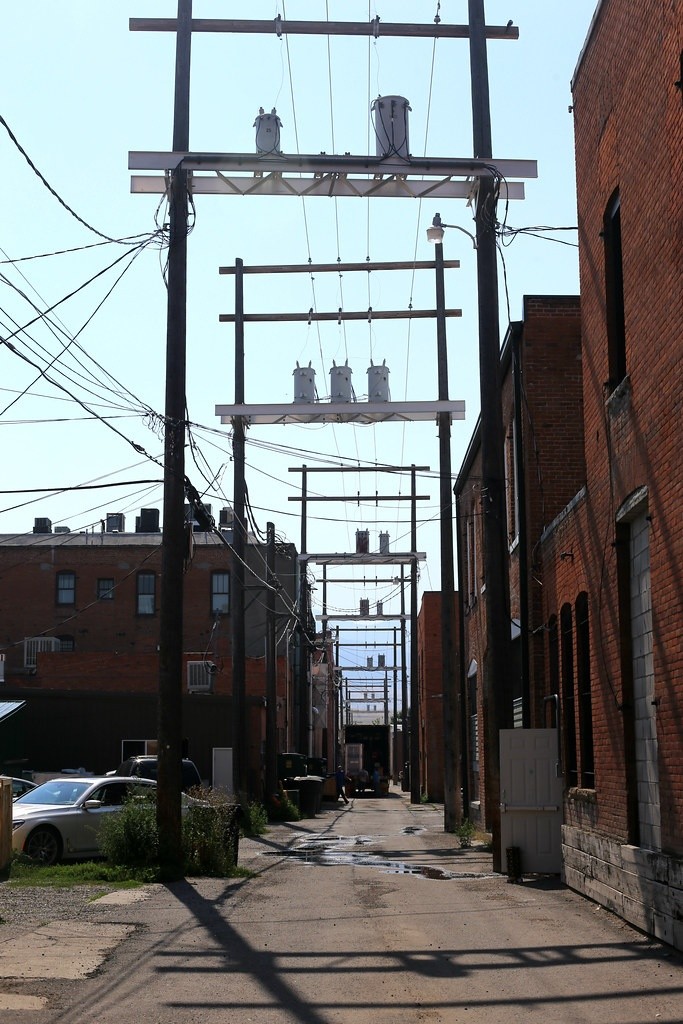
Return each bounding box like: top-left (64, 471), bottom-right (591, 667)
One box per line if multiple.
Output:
top-left (104, 755), bottom-right (201, 805)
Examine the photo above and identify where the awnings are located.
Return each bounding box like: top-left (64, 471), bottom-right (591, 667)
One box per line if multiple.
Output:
top-left (0, 699), bottom-right (26, 723)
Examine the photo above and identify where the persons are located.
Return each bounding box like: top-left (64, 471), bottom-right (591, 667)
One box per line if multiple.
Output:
top-left (335, 763), bottom-right (381, 804)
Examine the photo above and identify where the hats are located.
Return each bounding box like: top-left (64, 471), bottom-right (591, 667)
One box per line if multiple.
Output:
top-left (338, 766), bottom-right (341, 768)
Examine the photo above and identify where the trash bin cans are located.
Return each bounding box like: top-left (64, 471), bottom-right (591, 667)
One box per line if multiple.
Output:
top-left (0, 776), bottom-right (13, 880)
top-left (190, 803), bottom-right (241, 877)
top-left (294, 775), bottom-right (325, 819)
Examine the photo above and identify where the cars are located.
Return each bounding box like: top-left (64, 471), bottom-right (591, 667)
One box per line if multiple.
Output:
top-left (0, 774), bottom-right (58, 804)
top-left (11, 774), bottom-right (209, 867)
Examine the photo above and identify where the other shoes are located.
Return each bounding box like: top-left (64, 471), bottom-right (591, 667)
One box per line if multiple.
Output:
top-left (346, 801), bottom-right (349, 804)
